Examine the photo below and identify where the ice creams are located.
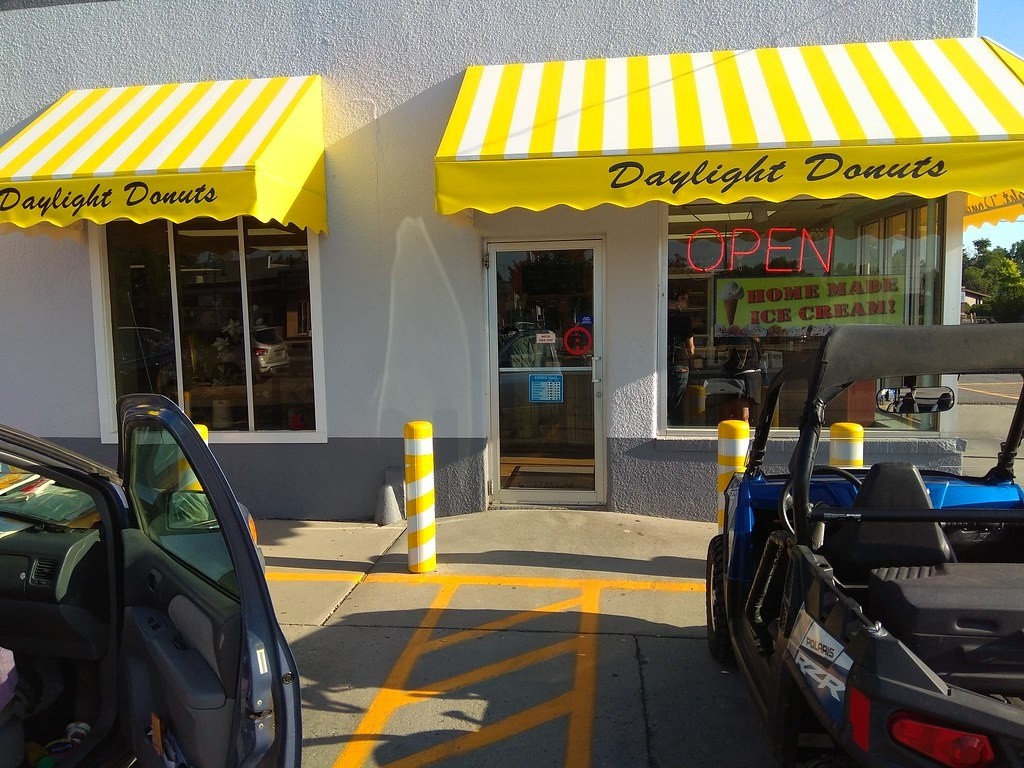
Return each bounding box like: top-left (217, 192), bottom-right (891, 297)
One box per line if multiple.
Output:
top-left (720, 281), bottom-right (745, 325)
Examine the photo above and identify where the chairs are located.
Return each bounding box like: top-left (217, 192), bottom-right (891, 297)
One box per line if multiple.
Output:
top-left (743, 462), bottom-right (954, 656)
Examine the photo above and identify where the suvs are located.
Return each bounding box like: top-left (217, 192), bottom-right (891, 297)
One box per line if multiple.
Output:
top-left (177, 324), bottom-right (290, 387)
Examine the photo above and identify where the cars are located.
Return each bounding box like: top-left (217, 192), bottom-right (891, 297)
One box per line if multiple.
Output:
top-left (0, 394), bottom-right (303, 768)
top-left (886, 393), bottom-right (953, 413)
top-left (115, 325), bottom-right (177, 396)
top-left (683, 336), bottom-right (767, 418)
top-left (704, 321), bottom-right (1024, 768)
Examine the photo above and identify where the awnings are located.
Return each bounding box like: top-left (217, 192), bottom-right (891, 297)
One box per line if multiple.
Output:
top-left (434, 33), bottom-right (1024, 215)
top-left (0, 74), bottom-right (326, 239)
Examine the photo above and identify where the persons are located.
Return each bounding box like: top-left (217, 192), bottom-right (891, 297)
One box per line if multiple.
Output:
top-left (722, 336), bottom-right (767, 427)
top-left (666, 285), bottom-right (694, 417)
